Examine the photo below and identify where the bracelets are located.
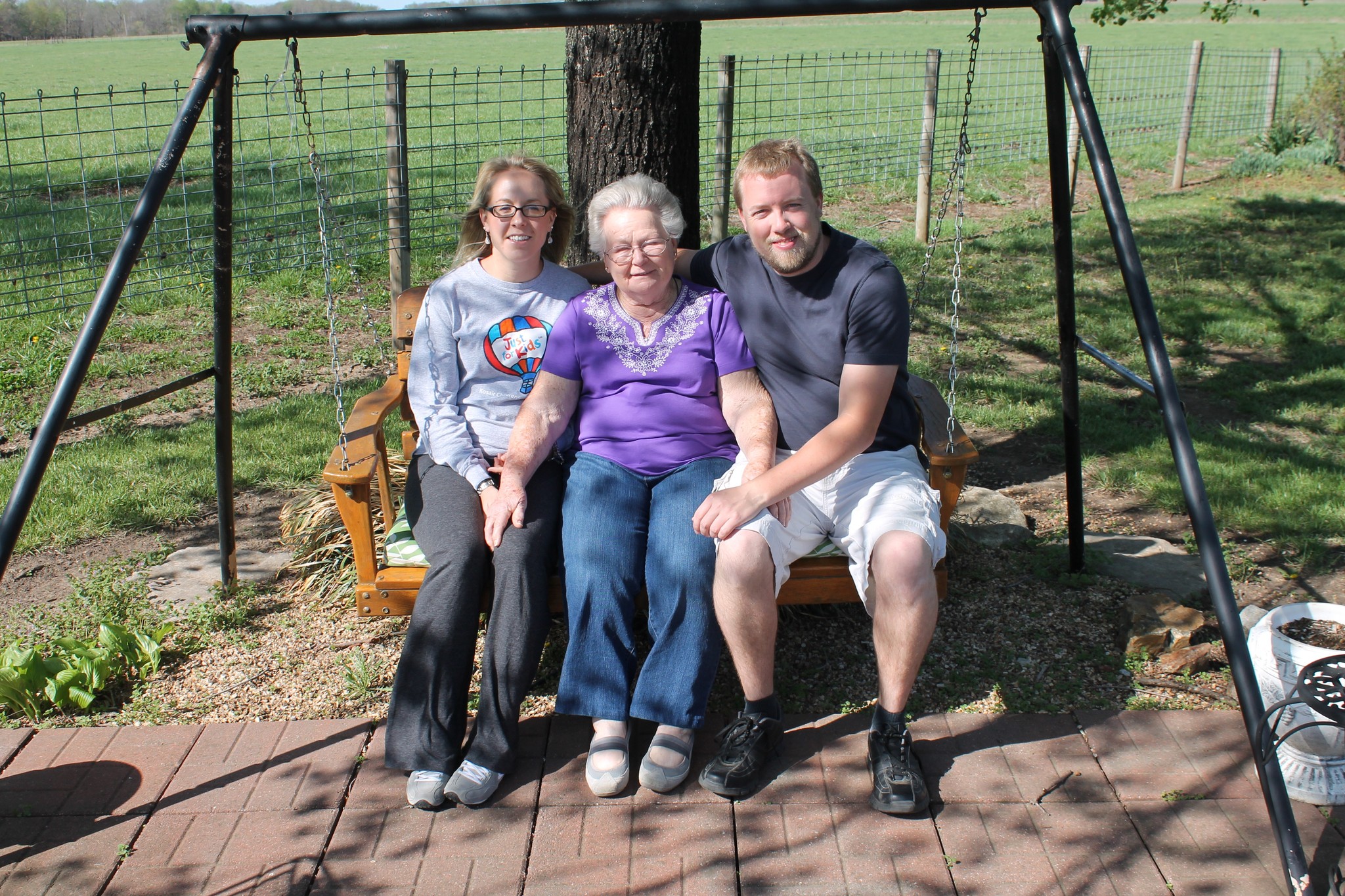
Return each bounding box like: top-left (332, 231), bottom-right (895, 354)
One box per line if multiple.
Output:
top-left (476, 477), bottom-right (494, 500)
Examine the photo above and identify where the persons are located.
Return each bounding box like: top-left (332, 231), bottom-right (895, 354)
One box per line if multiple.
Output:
top-left (383, 154), bottom-right (595, 810)
top-left (567, 139), bottom-right (947, 815)
top-left (484, 168), bottom-right (778, 799)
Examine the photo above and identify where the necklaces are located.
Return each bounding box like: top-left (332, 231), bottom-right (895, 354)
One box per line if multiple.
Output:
top-left (674, 277), bottom-right (681, 298)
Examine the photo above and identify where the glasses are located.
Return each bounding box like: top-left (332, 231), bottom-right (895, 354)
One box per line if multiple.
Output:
top-left (484, 204), bottom-right (551, 218)
top-left (603, 232), bottom-right (670, 263)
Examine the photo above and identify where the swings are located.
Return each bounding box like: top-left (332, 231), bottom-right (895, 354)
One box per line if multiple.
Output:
top-left (287, 9), bottom-right (986, 618)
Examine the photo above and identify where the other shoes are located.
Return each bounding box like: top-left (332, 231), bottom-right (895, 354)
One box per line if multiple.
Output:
top-left (585, 720), bottom-right (632, 796)
top-left (639, 729), bottom-right (695, 792)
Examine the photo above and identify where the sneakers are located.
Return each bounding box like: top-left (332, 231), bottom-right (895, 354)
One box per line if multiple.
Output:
top-left (406, 770), bottom-right (451, 809)
top-left (699, 701), bottom-right (782, 796)
top-left (444, 761), bottom-right (504, 807)
top-left (867, 728), bottom-right (929, 813)
